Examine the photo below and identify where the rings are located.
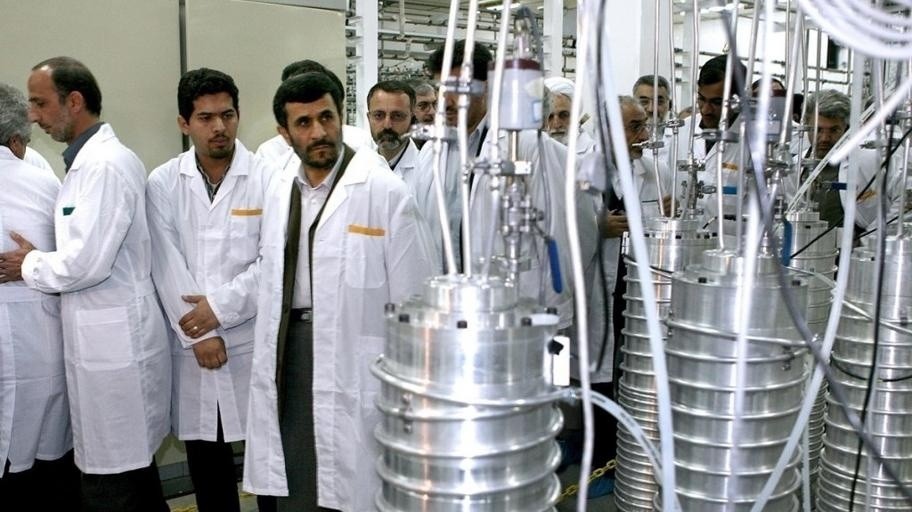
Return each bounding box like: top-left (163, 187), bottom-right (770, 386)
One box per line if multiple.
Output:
top-left (193, 326), bottom-right (200, 333)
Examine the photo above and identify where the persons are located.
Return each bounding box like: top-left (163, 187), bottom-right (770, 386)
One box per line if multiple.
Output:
top-left (1, 86), bottom-right (72, 511)
top-left (238, 69), bottom-right (432, 510)
top-left (1, 56), bottom-right (175, 512)
top-left (145, 65), bottom-right (283, 511)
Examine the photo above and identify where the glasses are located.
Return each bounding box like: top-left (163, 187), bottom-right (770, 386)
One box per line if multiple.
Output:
top-left (418, 101), bottom-right (439, 112)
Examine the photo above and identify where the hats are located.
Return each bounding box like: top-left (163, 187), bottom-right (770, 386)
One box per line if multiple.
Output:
top-left (541, 76), bottom-right (584, 119)
